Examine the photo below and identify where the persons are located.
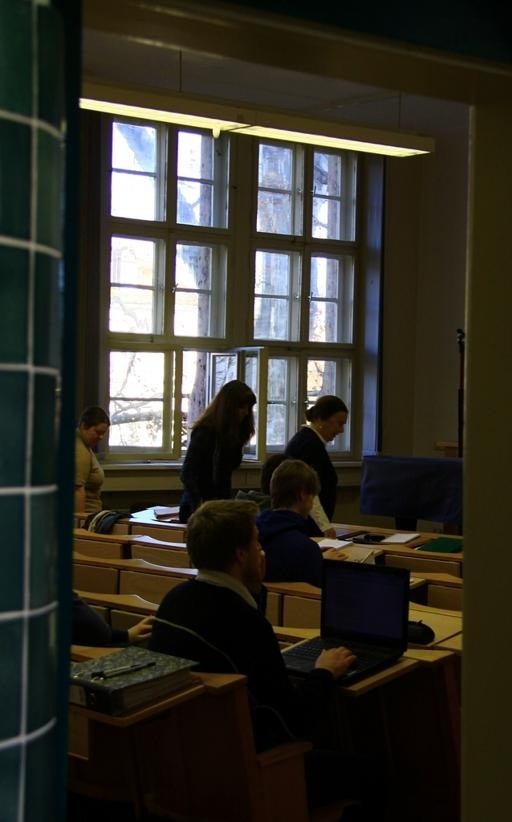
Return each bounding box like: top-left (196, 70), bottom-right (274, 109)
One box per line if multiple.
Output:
top-left (71, 588), bottom-right (156, 648)
top-left (74, 405), bottom-right (111, 513)
top-left (147, 498), bottom-right (358, 750)
top-left (257, 459), bottom-right (349, 589)
top-left (234, 454), bottom-right (294, 511)
top-left (284, 394), bottom-right (350, 539)
top-left (178, 379), bottom-right (258, 524)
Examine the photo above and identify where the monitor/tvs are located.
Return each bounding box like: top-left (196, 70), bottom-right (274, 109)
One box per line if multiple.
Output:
top-left (360, 455), bottom-right (463, 531)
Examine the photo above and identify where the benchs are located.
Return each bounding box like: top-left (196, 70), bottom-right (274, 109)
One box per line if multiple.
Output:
top-left (72, 498), bottom-right (468, 821)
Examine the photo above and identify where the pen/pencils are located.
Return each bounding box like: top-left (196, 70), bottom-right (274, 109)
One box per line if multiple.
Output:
top-left (90, 660), bottom-right (155, 681)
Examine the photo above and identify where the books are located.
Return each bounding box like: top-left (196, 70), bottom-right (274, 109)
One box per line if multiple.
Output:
top-left (315, 537), bottom-right (374, 563)
top-left (153, 505), bottom-right (180, 519)
top-left (381, 532), bottom-right (420, 544)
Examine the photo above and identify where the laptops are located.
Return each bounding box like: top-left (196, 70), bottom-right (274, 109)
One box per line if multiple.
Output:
top-left (281, 558), bottom-right (411, 686)
